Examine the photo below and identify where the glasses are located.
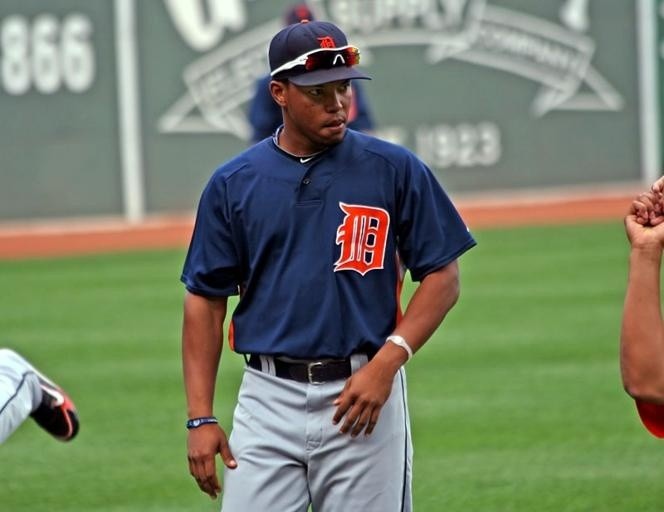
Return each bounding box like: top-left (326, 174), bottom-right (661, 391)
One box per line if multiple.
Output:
top-left (271, 45), bottom-right (360, 77)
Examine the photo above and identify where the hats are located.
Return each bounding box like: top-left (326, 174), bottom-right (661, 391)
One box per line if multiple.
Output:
top-left (268, 20), bottom-right (371, 87)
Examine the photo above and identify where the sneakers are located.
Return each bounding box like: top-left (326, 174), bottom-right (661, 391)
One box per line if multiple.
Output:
top-left (1, 349), bottom-right (79, 441)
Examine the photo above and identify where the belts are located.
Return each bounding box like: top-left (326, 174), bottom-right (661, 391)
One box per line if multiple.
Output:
top-left (248, 351), bottom-right (377, 383)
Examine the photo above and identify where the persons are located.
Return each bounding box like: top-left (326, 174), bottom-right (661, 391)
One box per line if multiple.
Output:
top-left (247, 2), bottom-right (379, 145)
top-left (0, 342), bottom-right (80, 458)
top-left (175, 18), bottom-right (476, 512)
top-left (617, 171), bottom-right (664, 444)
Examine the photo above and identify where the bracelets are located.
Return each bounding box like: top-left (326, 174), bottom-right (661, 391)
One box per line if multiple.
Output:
top-left (384, 336), bottom-right (413, 368)
top-left (183, 415), bottom-right (219, 431)
